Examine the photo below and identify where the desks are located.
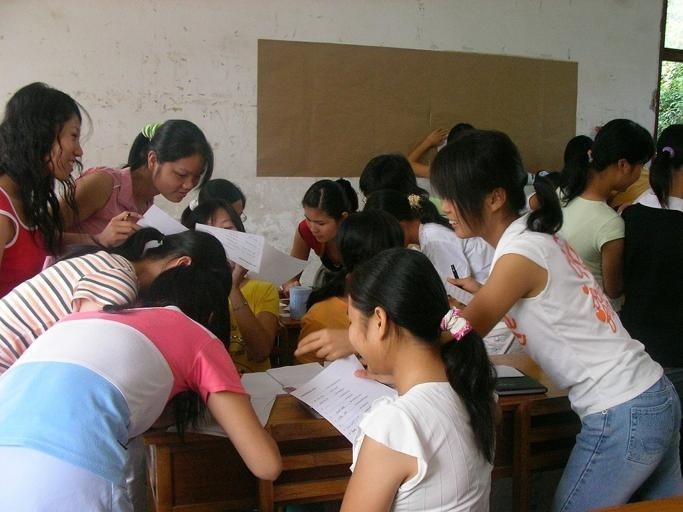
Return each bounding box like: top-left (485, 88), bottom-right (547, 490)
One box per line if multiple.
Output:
top-left (145, 353), bottom-right (682, 512)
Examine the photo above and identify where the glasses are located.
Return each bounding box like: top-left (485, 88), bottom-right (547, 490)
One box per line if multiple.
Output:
top-left (238, 211), bottom-right (248, 223)
top-left (362, 197), bottom-right (369, 204)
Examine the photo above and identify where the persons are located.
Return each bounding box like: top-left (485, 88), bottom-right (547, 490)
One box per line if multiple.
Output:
top-left (0, 262), bottom-right (284, 512)
top-left (278, 118), bottom-right (681, 398)
top-left (1, 81), bottom-right (94, 301)
top-left (0, 121), bottom-right (280, 374)
top-left (338, 247), bottom-right (502, 511)
top-left (291, 127), bottom-right (682, 511)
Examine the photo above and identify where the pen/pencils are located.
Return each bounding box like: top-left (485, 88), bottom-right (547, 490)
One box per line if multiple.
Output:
top-left (122, 212), bottom-right (131, 221)
top-left (451, 264), bottom-right (459, 279)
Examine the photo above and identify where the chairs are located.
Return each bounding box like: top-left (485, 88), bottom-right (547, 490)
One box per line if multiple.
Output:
top-left (260, 420), bottom-right (352, 512)
top-left (515, 401), bottom-right (581, 511)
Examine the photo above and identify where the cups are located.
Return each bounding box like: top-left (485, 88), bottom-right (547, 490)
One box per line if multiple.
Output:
top-left (288, 285), bottom-right (313, 320)
